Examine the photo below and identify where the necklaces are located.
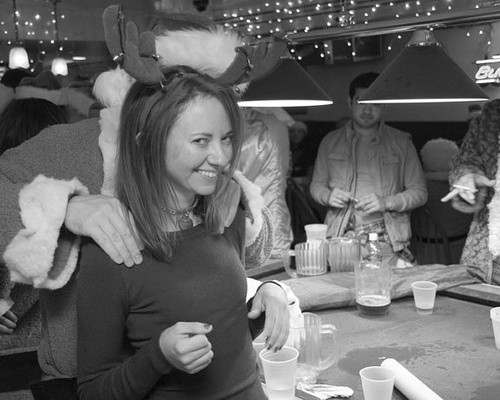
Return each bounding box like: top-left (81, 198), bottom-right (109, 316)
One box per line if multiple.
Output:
top-left (160, 194), bottom-right (199, 230)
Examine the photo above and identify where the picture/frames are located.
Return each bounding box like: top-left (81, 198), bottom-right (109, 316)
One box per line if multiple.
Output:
top-left (352, 36), bottom-right (385, 61)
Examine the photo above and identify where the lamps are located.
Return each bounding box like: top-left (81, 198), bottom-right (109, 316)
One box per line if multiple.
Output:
top-left (51, 0), bottom-right (69, 76)
top-left (235, 36), bottom-right (333, 107)
top-left (8, 0), bottom-right (30, 69)
top-left (356, 29), bottom-right (490, 104)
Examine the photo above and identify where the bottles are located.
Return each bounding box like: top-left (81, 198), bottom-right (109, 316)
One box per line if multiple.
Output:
top-left (304, 223), bottom-right (328, 249)
top-left (364, 232), bottom-right (392, 266)
top-left (353, 259), bottom-right (392, 318)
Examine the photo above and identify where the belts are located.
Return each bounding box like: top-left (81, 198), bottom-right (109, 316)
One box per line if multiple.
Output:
top-left (417, 233), bottom-right (467, 244)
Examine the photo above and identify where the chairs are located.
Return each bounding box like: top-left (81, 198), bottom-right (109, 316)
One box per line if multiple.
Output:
top-left (409, 206), bottom-right (452, 265)
top-left (287, 177), bottom-right (321, 244)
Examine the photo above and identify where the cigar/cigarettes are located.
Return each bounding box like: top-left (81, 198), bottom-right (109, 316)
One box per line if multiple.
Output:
top-left (452, 185), bottom-right (478, 193)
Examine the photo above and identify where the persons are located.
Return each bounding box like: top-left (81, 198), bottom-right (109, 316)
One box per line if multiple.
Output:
top-left (441, 98), bottom-right (500, 286)
top-left (310, 73), bottom-right (428, 252)
top-left (0, 0), bottom-right (308, 400)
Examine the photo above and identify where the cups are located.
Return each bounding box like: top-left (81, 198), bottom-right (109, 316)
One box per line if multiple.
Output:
top-left (490, 307), bottom-right (500, 350)
top-left (411, 280), bottom-right (437, 316)
top-left (259, 346), bottom-right (299, 399)
top-left (291, 310), bottom-right (340, 388)
top-left (324, 237), bottom-right (361, 271)
top-left (283, 240), bottom-right (327, 280)
top-left (359, 366), bottom-right (396, 400)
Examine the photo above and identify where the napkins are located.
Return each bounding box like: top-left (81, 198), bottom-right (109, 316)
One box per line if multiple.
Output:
top-left (297, 381), bottom-right (354, 400)
top-left (380, 358), bottom-right (443, 400)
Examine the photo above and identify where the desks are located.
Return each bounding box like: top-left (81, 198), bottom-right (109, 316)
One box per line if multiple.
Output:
top-left (252, 263), bottom-right (500, 400)
